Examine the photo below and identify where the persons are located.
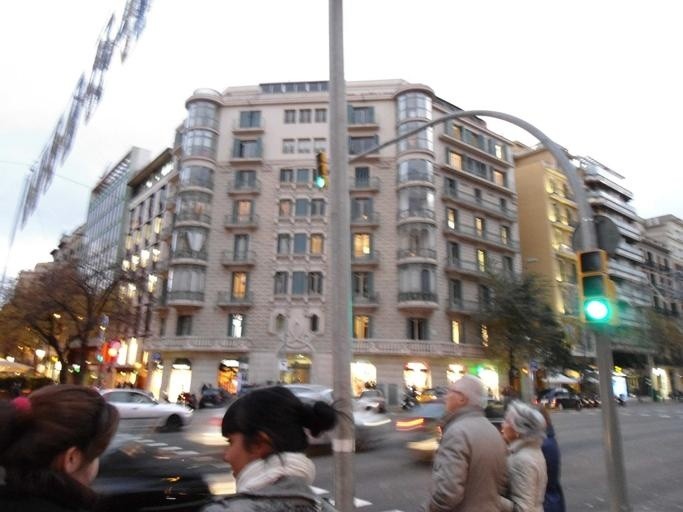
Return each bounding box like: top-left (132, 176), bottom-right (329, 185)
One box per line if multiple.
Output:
top-left (204, 386), bottom-right (340, 512)
top-left (428, 375), bottom-right (566, 512)
top-left (0, 383), bottom-right (119, 512)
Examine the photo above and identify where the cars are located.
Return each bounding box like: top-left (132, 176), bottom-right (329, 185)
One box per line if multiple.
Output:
top-left (280, 383), bottom-right (338, 408)
top-left (531, 386), bottom-right (583, 411)
top-left (198, 388), bottom-right (234, 409)
top-left (99, 388), bottom-right (196, 433)
top-left (359, 389), bottom-right (385, 413)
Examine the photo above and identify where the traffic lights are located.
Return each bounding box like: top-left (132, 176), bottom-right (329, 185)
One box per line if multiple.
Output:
top-left (315, 152), bottom-right (329, 189)
top-left (575, 249), bottom-right (621, 326)
